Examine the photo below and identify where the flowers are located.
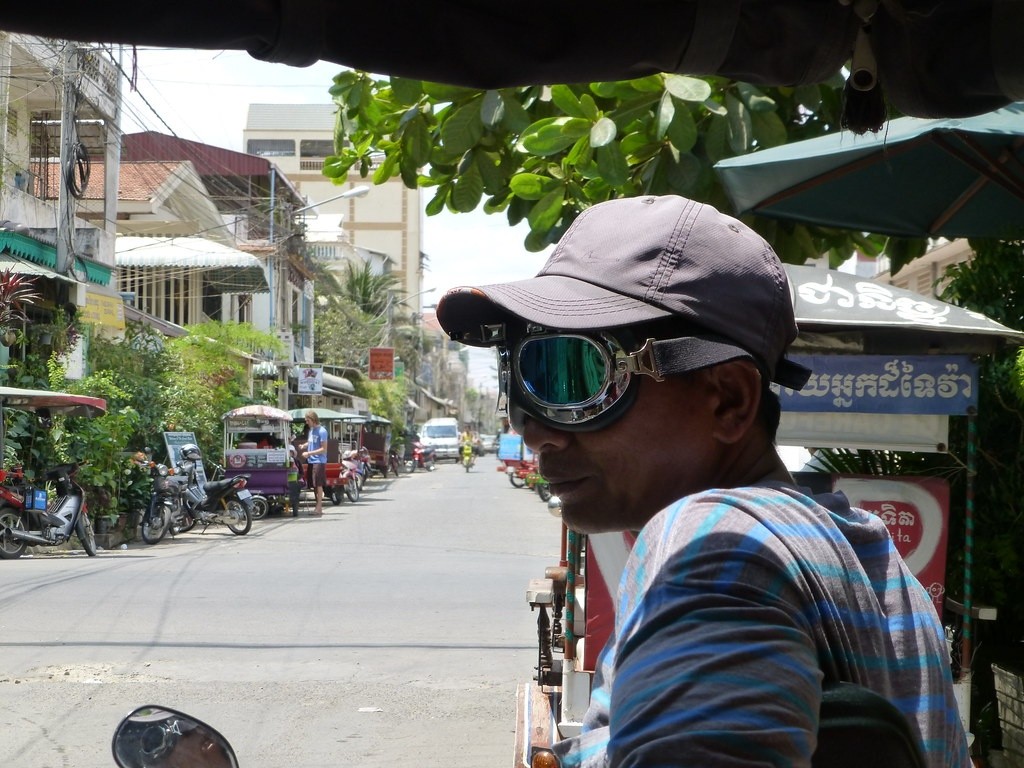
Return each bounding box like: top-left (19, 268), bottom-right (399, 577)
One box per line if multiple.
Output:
top-left (116, 453), bottom-right (154, 510)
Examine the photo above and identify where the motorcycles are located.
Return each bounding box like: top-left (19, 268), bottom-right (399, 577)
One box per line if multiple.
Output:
top-left (133, 444), bottom-right (254, 545)
top-left (220, 404), bottom-right (294, 521)
top-left (0, 460), bottom-right (97, 560)
top-left (408, 442), bottom-right (437, 474)
top-left (285, 408), bottom-right (393, 505)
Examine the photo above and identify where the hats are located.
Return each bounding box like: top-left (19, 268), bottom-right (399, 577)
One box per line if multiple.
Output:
top-left (436, 193), bottom-right (813, 392)
top-left (289, 444), bottom-right (297, 458)
top-left (127, 706), bottom-right (175, 723)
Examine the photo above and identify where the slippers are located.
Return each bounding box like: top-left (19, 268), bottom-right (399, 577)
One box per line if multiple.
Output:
top-left (308, 510), bottom-right (324, 515)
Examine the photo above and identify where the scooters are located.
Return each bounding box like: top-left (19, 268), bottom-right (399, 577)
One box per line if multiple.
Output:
top-left (459, 440), bottom-right (475, 473)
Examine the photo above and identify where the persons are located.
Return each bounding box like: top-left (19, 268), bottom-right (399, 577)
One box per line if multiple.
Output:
top-left (435, 195), bottom-right (973, 768)
top-left (459, 424), bottom-right (480, 466)
top-left (290, 411), bottom-right (328, 516)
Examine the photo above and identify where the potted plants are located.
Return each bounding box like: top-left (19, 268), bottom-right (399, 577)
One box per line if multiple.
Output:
top-left (91, 472), bottom-right (112, 534)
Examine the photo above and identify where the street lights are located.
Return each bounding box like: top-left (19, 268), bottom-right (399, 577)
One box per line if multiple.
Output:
top-left (276, 185), bottom-right (370, 406)
top-left (386, 286), bottom-right (438, 410)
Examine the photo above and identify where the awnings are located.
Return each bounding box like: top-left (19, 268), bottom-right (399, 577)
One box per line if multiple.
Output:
top-left (115, 235), bottom-right (271, 297)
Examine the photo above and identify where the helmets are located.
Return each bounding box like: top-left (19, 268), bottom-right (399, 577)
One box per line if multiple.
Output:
top-left (180, 444), bottom-right (203, 460)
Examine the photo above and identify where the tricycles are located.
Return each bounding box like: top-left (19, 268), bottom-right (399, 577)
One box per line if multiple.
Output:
top-left (497, 432), bottom-right (551, 502)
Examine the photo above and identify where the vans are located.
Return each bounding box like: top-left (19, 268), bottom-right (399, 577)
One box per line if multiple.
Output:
top-left (419, 417), bottom-right (460, 464)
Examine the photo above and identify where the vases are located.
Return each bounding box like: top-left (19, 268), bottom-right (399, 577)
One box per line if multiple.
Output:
top-left (118, 512), bottom-right (128, 529)
top-left (126, 509), bottom-right (141, 527)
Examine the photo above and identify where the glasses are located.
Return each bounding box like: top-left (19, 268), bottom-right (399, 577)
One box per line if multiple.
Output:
top-left (139, 719), bottom-right (199, 768)
top-left (496, 334), bottom-right (667, 433)
